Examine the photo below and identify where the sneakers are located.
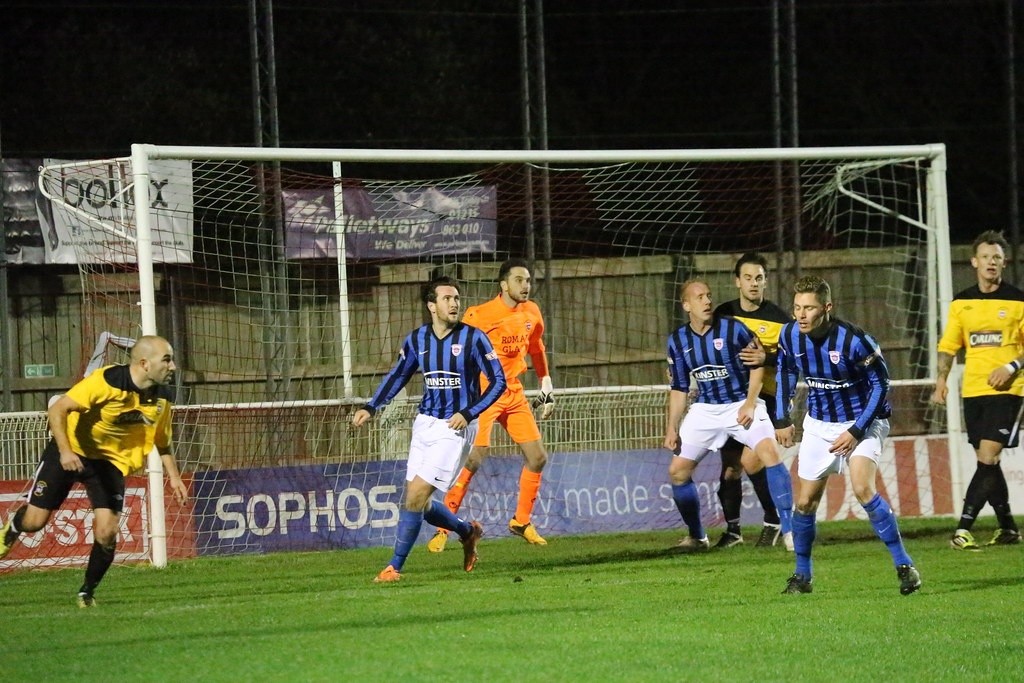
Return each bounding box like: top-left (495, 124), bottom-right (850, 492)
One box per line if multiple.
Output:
top-left (983, 528), bottom-right (1022, 546)
top-left (0, 512), bottom-right (23, 560)
top-left (708, 531), bottom-right (744, 552)
top-left (374, 564), bottom-right (401, 582)
top-left (78, 591), bottom-right (96, 609)
top-left (780, 573), bottom-right (813, 594)
top-left (950, 530), bottom-right (981, 552)
top-left (670, 533), bottom-right (710, 554)
top-left (754, 525), bottom-right (782, 547)
top-left (459, 521), bottom-right (485, 572)
top-left (508, 515), bottom-right (548, 546)
top-left (896, 564), bottom-right (921, 595)
top-left (427, 530), bottom-right (449, 553)
top-left (783, 530), bottom-right (795, 551)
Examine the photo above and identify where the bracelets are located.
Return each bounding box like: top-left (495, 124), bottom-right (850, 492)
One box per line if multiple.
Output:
top-left (1005, 359), bottom-right (1022, 376)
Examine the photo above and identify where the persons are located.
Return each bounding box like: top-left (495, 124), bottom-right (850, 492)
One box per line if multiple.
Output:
top-left (0, 336), bottom-right (190, 611)
top-left (427, 258), bottom-right (556, 552)
top-left (661, 251), bottom-right (798, 552)
top-left (351, 275), bottom-right (509, 582)
top-left (775, 276), bottom-right (923, 595)
top-left (933, 229), bottom-right (1024, 551)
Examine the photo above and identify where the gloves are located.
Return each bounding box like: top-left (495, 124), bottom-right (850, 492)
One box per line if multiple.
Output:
top-left (531, 375), bottom-right (556, 419)
